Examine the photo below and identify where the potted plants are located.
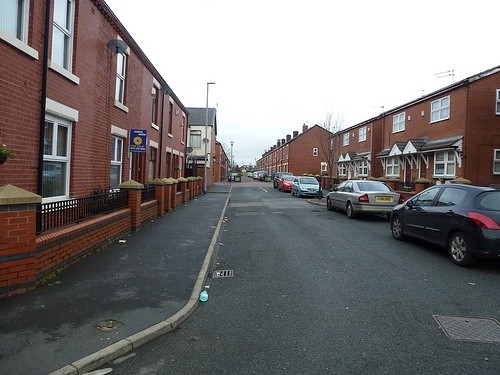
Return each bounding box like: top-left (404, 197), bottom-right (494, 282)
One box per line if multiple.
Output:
top-left (0, 144), bottom-right (18, 164)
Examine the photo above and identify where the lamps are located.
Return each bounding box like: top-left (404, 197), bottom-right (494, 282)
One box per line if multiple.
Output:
top-left (107, 39), bottom-right (127, 53)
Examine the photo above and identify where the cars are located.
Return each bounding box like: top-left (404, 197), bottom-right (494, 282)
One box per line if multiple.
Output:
top-left (327, 179), bottom-right (400, 221)
top-left (228, 173), bottom-right (239, 180)
top-left (247, 171), bottom-right (267, 181)
top-left (292, 176), bottom-right (325, 199)
top-left (388, 182), bottom-right (500, 266)
top-left (278, 175), bottom-right (292, 190)
top-left (273, 172), bottom-right (293, 189)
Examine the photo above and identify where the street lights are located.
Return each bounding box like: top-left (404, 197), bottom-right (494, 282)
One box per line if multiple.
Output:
top-left (203, 81), bottom-right (215, 191)
top-left (230, 141), bottom-right (234, 173)
